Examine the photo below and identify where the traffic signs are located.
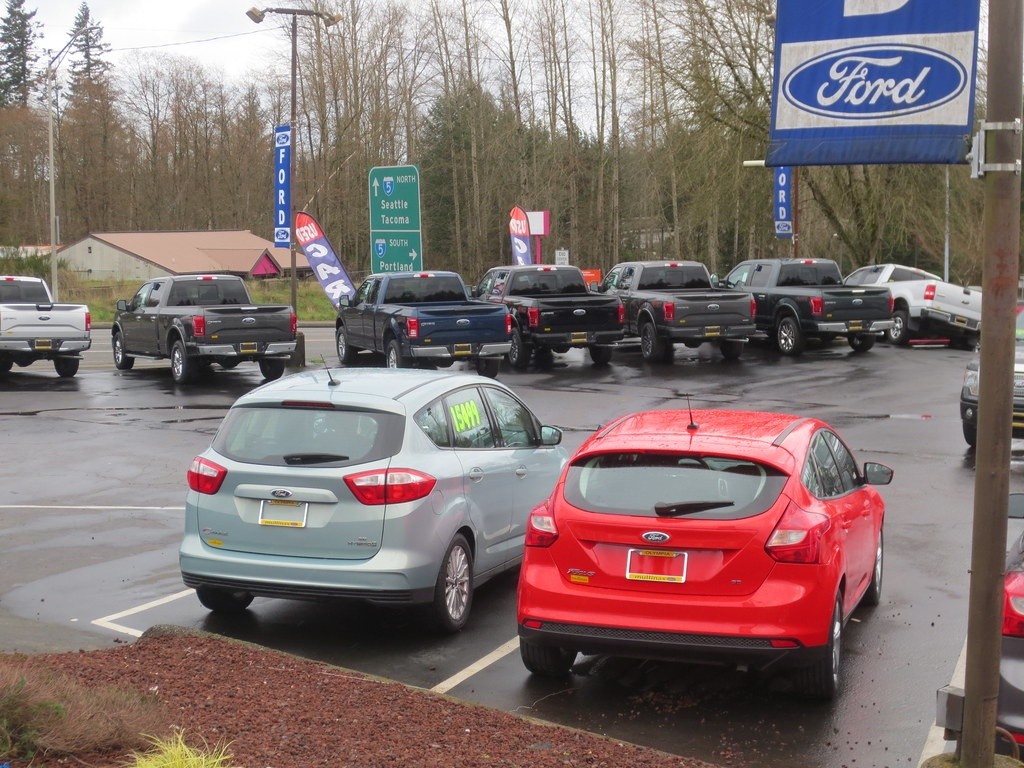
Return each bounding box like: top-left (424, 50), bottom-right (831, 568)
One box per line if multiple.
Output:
top-left (370, 230), bottom-right (423, 275)
top-left (367, 165), bottom-right (420, 232)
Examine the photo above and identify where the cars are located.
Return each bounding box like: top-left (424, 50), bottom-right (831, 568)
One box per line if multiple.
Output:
top-left (992, 493), bottom-right (1023, 764)
top-left (178, 367), bottom-right (574, 632)
top-left (518, 410), bottom-right (893, 697)
top-left (960, 302), bottom-right (1024, 446)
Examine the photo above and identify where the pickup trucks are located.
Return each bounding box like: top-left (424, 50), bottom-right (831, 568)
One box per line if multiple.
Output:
top-left (589, 261), bottom-right (756, 364)
top-left (334, 270), bottom-right (512, 378)
top-left (842, 264), bottom-right (982, 351)
top-left (0, 276), bottom-right (92, 377)
top-left (111, 275), bottom-right (297, 384)
top-left (720, 258), bottom-right (895, 354)
top-left (466, 265), bottom-right (624, 370)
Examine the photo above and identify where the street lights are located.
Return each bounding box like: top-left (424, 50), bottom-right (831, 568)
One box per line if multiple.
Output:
top-left (246, 7), bottom-right (344, 366)
top-left (47, 26), bottom-right (99, 302)
